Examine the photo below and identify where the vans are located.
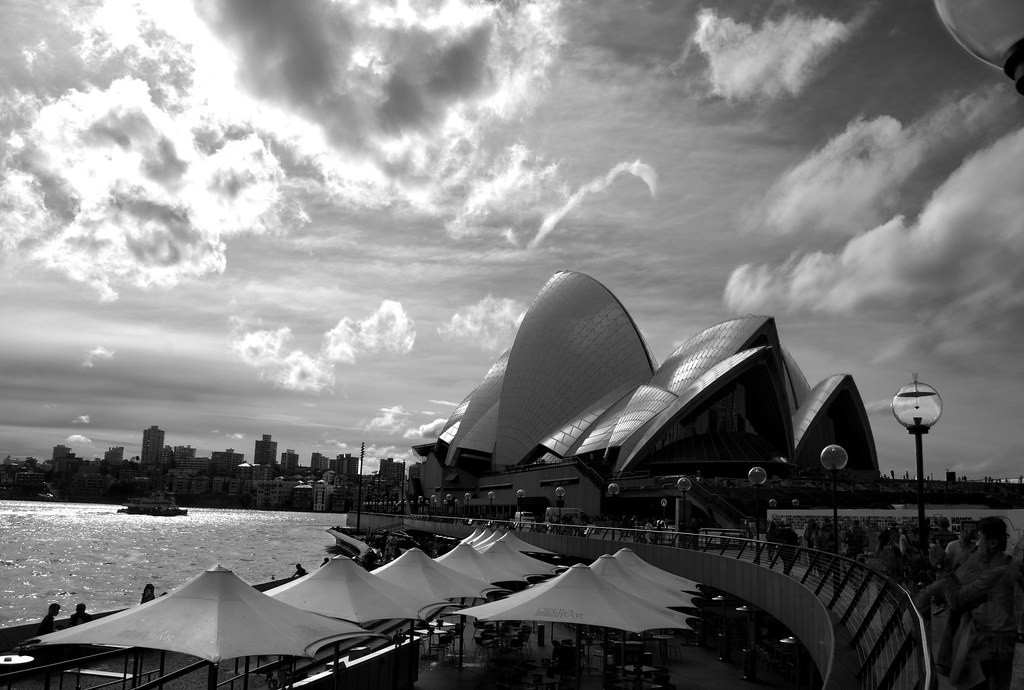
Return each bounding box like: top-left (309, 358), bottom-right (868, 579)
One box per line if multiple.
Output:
top-left (546, 507), bottom-right (590, 528)
top-left (515, 511), bottom-right (537, 525)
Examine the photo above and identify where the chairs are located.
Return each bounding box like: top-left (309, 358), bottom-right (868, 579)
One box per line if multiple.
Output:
top-left (424, 620), bottom-right (683, 690)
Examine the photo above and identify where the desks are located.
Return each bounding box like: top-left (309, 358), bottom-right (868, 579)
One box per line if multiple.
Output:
top-left (520, 678), bottom-right (561, 690)
top-left (0, 654), bottom-right (35, 690)
top-left (428, 619), bottom-right (456, 641)
top-left (572, 638), bottom-right (603, 673)
top-left (614, 640), bottom-right (645, 663)
top-left (649, 635), bottom-right (675, 659)
top-left (616, 664), bottom-right (660, 682)
top-left (414, 628), bottom-right (448, 655)
top-left (611, 681), bottom-right (663, 690)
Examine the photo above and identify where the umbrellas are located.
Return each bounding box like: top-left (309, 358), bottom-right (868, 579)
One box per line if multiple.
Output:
top-left (369, 546), bottom-right (510, 669)
top-left (261, 554), bottom-right (461, 690)
top-left (26, 562), bottom-right (387, 690)
top-left (440, 545), bottom-right (705, 690)
top-left (433, 526), bottom-right (569, 603)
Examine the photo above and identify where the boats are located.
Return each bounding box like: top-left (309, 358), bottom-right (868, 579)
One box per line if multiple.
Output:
top-left (117, 487), bottom-right (190, 515)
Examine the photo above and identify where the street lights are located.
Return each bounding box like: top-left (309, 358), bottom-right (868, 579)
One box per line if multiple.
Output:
top-left (443, 499), bottom-right (447, 515)
top-left (454, 499), bottom-right (460, 515)
top-left (677, 477), bottom-right (691, 547)
top-left (431, 495), bottom-right (436, 516)
top-left (555, 487), bottom-right (565, 535)
top-left (608, 484), bottom-right (620, 540)
top-left (746, 467), bottom-right (769, 565)
top-left (821, 444), bottom-right (849, 553)
top-left (446, 493), bottom-right (452, 516)
top-left (517, 489), bottom-right (526, 530)
top-left (488, 491), bottom-right (495, 522)
top-left (891, 381), bottom-right (943, 586)
top-left (464, 493), bottom-right (472, 518)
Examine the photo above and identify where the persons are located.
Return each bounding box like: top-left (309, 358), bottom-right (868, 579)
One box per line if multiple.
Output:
top-left (71, 603), bottom-right (94, 626)
top-left (622, 516), bottom-right (1024, 690)
top-left (142, 584), bottom-right (154, 603)
top-left (321, 558), bottom-right (329, 565)
top-left (292, 564), bottom-right (307, 577)
top-left (331, 526), bottom-right (402, 570)
top-left (37, 604), bottom-right (60, 636)
